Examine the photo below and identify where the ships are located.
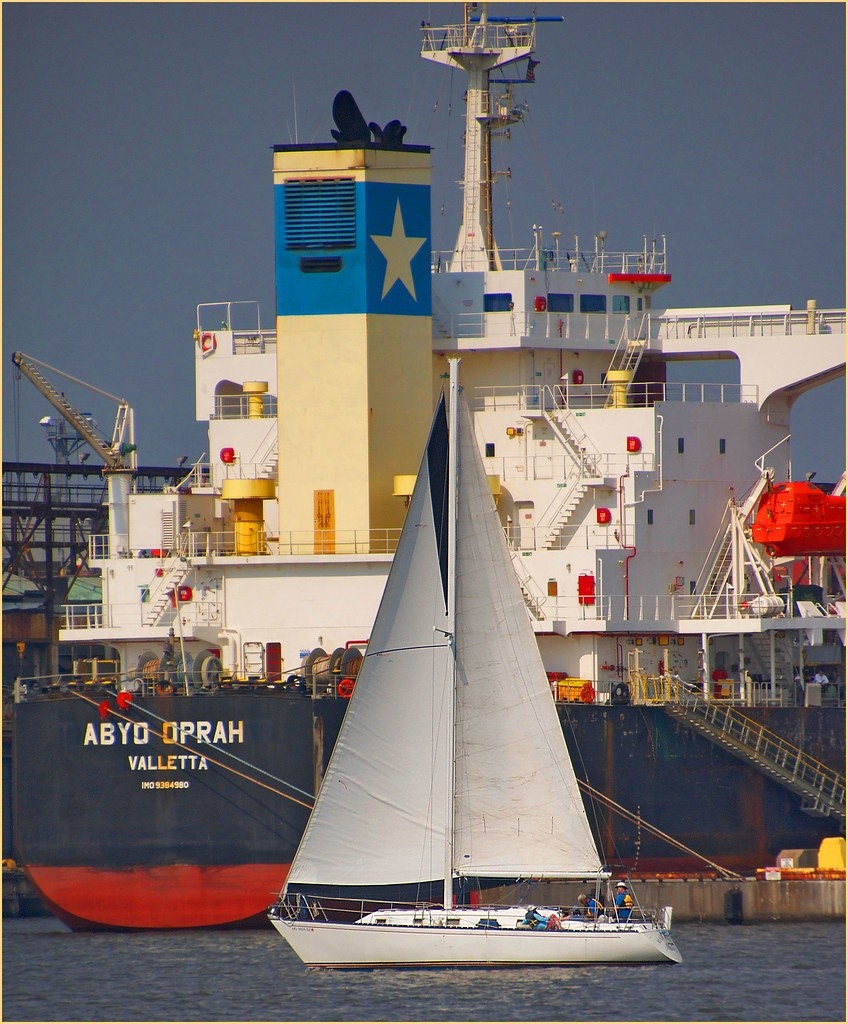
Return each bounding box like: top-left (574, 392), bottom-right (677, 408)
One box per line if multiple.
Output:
top-left (15, 0), bottom-right (844, 930)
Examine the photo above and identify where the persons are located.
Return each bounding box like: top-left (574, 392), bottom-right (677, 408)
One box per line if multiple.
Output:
top-left (712, 665), bottom-right (728, 699)
top-left (813, 669), bottom-right (829, 686)
top-left (612, 882), bottom-right (634, 923)
top-left (577, 888), bottom-right (608, 920)
top-left (522, 905), bottom-right (564, 931)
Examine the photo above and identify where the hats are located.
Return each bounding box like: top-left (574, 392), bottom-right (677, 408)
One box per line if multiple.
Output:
top-left (614, 882), bottom-right (630, 889)
top-left (527, 905), bottom-right (538, 912)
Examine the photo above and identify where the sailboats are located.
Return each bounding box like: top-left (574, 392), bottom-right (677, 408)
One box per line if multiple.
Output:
top-left (268, 354), bottom-right (685, 969)
